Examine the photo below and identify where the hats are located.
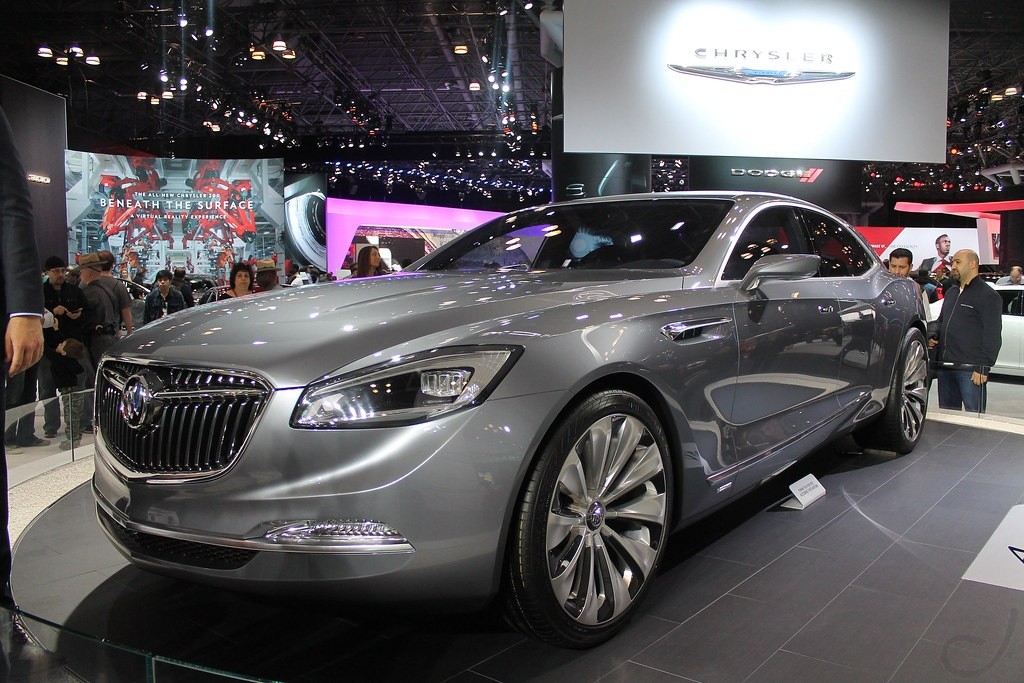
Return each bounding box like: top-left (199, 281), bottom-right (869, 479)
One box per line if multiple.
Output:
top-left (42, 256), bottom-right (66, 271)
top-left (75, 253), bottom-right (108, 271)
top-left (255, 259), bottom-right (281, 273)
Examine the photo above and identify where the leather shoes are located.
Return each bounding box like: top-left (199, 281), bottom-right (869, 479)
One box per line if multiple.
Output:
top-left (0, 606), bottom-right (33, 646)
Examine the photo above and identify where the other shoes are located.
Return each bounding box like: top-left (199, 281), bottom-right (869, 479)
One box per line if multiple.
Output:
top-left (44, 429), bottom-right (57, 438)
top-left (6, 434), bottom-right (50, 447)
top-left (80, 425), bottom-right (94, 434)
top-left (59, 439), bottom-right (80, 449)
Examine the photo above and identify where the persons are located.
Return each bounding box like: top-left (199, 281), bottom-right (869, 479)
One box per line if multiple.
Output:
top-left (996, 266), bottom-right (1023, 285)
top-left (887, 248), bottom-right (913, 281)
top-left (0, 112), bottom-right (45, 677)
top-left (928, 248), bottom-right (1002, 413)
top-left (2, 248), bottom-right (195, 451)
top-left (917, 233), bottom-right (955, 278)
top-left (343, 245), bottom-right (387, 277)
top-left (286, 264), bottom-right (338, 285)
top-left (219, 259), bottom-right (285, 300)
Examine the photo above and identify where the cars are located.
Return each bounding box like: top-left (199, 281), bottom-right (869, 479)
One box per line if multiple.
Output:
top-left (91, 197), bottom-right (931, 650)
top-left (922, 286), bottom-right (1024, 377)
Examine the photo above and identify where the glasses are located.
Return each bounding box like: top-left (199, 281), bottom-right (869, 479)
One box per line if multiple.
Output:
top-left (371, 254), bottom-right (380, 257)
top-left (1010, 276), bottom-right (1021, 280)
top-left (50, 269), bottom-right (66, 274)
